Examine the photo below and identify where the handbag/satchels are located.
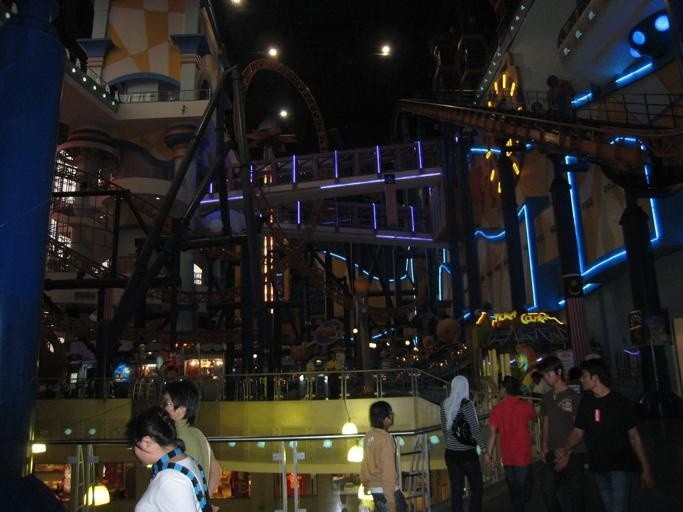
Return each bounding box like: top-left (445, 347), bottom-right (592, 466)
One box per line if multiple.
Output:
top-left (451, 400), bottom-right (480, 447)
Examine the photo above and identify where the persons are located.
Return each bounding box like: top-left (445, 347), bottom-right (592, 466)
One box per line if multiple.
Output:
top-left (545, 76), bottom-right (575, 112)
top-left (488, 375), bottom-right (539, 511)
top-left (156, 377), bottom-right (221, 500)
top-left (123, 407), bottom-right (215, 512)
top-left (553, 358), bottom-right (655, 512)
top-left (535, 354), bottom-right (586, 512)
top-left (494, 362), bottom-right (582, 400)
top-left (439, 373), bottom-right (486, 510)
top-left (359, 399), bottom-right (407, 511)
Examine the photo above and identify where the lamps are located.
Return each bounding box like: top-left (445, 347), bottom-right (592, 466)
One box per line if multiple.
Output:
top-left (81, 480), bottom-right (111, 507)
top-left (341, 375), bottom-right (364, 463)
